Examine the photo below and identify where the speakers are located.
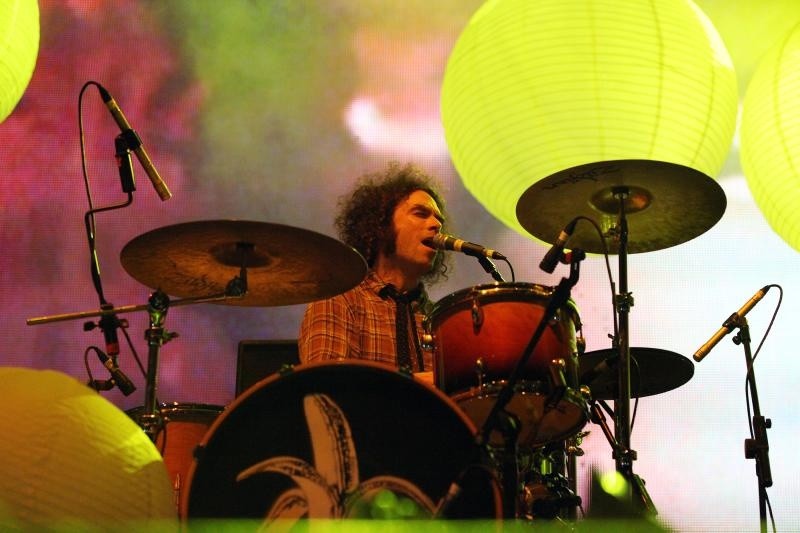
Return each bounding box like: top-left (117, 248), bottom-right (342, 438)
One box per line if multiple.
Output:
top-left (234, 340), bottom-right (302, 400)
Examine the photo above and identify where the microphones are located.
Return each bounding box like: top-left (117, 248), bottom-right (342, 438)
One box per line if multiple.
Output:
top-left (694, 284), bottom-right (771, 362)
top-left (433, 233), bottom-right (508, 261)
top-left (99, 83), bottom-right (172, 201)
top-left (539, 219), bottom-right (578, 275)
top-left (94, 347), bottom-right (136, 397)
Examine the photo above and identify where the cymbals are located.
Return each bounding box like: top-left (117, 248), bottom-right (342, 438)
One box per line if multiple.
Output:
top-left (120, 219), bottom-right (368, 309)
top-left (573, 346), bottom-right (694, 397)
top-left (514, 157), bottom-right (728, 254)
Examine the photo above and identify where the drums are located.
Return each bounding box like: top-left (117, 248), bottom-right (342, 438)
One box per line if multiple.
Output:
top-left (506, 442), bottom-right (573, 502)
top-left (123, 398), bottom-right (229, 521)
top-left (424, 280), bottom-right (588, 436)
top-left (180, 360), bottom-right (503, 523)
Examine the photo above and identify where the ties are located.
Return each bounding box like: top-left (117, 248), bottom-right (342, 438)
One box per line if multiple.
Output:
top-left (381, 284), bottom-right (422, 376)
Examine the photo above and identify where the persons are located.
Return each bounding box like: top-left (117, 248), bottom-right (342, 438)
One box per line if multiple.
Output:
top-left (297, 163), bottom-right (448, 386)
